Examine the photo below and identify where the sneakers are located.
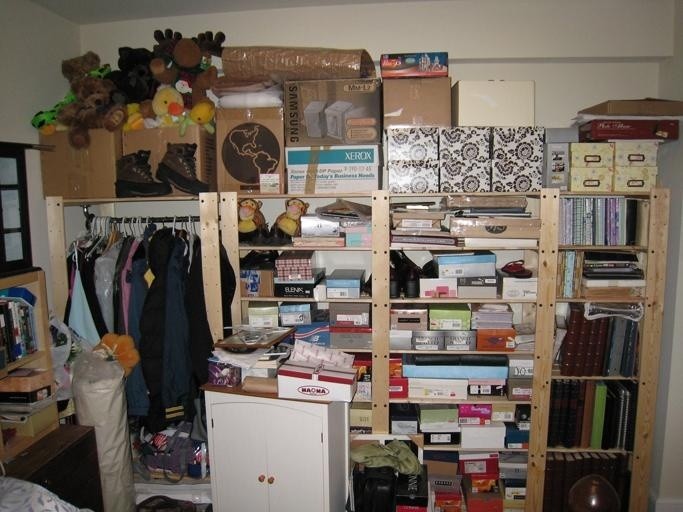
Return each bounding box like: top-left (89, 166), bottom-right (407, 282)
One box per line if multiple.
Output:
top-left (389, 266), bottom-right (404, 300)
top-left (401, 265), bottom-right (420, 299)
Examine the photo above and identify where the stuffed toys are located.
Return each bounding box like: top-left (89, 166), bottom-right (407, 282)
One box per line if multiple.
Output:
top-left (31, 28), bottom-right (224, 148)
top-left (275, 199), bottom-right (309, 237)
top-left (239, 198), bottom-right (266, 233)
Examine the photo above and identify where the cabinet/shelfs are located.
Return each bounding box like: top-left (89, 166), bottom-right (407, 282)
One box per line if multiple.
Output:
top-left (536, 186), bottom-right (669, 512)
top-left (40, 190), bottom-right (223, 490)
top-left (9, 422), bottom-right (104, 510)
top-left (0, 260), bottom-right (61, 471)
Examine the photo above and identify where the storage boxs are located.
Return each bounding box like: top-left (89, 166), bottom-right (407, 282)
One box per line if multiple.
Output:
top-left (208, 192), bottom-right (542, 512)
top-left (39, 51), bottom-right (682, 199)
top-left (0, 365), bottom-right (55, 401)
top-left (0, 401), bottom-right (60, 441)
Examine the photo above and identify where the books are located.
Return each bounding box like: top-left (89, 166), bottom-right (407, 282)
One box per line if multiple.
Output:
top-left (541, 197), bottom-right (649, 511)
top-left (0, 299), bottom-right (55, 426)
top-left (391, 196), bottom-right (540, 250)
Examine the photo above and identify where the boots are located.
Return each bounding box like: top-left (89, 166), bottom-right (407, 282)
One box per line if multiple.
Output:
top-left (114, 149), bottom-right (173, 197)
top-left (154, 142), bottom-right (211, 195)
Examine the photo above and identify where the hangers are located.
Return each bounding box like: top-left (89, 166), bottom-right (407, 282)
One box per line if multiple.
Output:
top-left (62, 205), bottom-right (198, 271)
